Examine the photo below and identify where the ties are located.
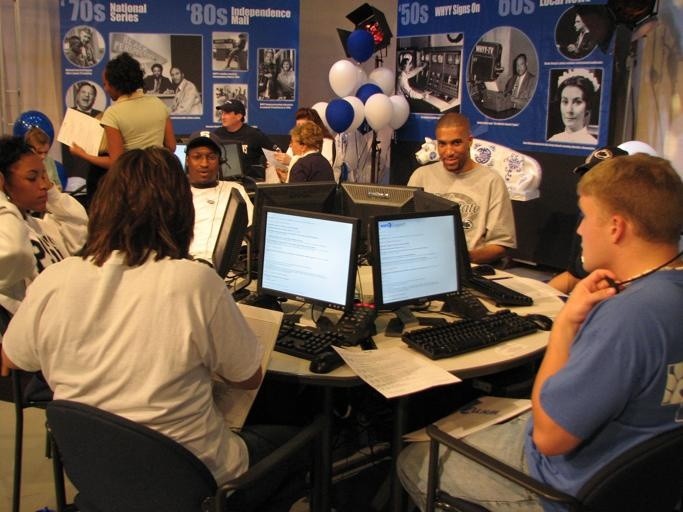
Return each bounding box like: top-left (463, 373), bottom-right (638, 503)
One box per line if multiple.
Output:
top-left (511, 76), bottom-right (521, 98)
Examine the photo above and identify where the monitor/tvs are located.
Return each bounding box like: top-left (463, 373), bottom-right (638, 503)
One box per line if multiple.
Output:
top-left (340, 182), bottom-right (423, 250)
top-left (208, 188), bottom-right (250, 302)
top-left (414, 190), bottom-right (472, 277)
top-left (172, 143), bottom-right (187, 171)
top-left (256, 205), bottom-right (361, 326)
top-left (217, 138), bottom-right (244, 180)
top-left (371, 210), bottom-right (461, 337)
top-left (253, 180), bottom-right (338, 276)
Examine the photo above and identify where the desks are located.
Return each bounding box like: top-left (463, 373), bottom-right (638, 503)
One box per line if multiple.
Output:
top-left (223, 257), bottom-right (575, 511)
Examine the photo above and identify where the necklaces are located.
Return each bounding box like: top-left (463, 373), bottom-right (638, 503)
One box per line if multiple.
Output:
top-left (614, 251), bottom-right (682, 286)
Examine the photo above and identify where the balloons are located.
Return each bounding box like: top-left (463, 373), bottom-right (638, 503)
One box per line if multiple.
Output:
top-left (310, 28), bottom-right (410, 140)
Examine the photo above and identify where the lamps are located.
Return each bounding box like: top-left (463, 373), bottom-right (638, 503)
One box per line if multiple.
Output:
top-left (571, 0), bottom-right (660, 152)
top-left (334, 1), bottom-right (393, 184)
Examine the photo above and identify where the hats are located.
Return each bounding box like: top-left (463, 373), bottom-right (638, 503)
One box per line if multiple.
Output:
top-left (216, 99), bottom-right (245, 112)
top-left (186, 131), bottom-right (223, 155)
top-left (574, 146), bottom-right (628, 175)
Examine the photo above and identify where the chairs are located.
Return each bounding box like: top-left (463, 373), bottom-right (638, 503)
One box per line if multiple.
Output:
top-left (42, 398), bottom-right (333, 512)
top-left (421, 421), bottom-right (681, 510)
top-left (0, 302), bottom-right (53, 510)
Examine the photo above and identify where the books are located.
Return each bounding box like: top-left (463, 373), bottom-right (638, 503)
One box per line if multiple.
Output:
top-left (401, 395), bottom-right (533, 443)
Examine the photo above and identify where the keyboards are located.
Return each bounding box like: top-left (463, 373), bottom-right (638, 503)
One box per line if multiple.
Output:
top-left (462, 277), bottom-right (532, 306)
top-left (274, 319), bottom-right (343, 360)
top-left (402, 312), bottom-right (537, 359)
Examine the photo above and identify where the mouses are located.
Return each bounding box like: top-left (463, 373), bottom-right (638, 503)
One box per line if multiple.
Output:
top-left (527, 313), bottom-right (552, 331)
top-left (310, 350), bottom-right (344, 373)
top-left (472, 265), bottom-right (495, 275)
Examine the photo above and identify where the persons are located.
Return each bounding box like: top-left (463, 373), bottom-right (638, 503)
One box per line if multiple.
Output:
top-left (0, 135), bottom-right (91, 316)
top-left (564, 10), bottom-right (598, 58)
top-left (213, 99), bottom-right (267, 178)
top-left (547, 144), bottom-right (628, 294)
top-left (504, 53), bottom-right (536, 110)
top-left (274, 107), bottom-right (334, 168)
top-left (68, 51), bottom-right (177, 170)
top-left (266, 120), bottom-right (334, 184)
top-left (548, 69), bottom-right (602, 144)
top-left (183, 128), bottom-right (254, 254)
top-left (71, 82), bottom-right (102, 118)
top-left (67, 35), bottom-right (85, 66)
top-left (143, 63), bottom-right (172, 94)
top-left (78, 28), bottom-right (98, 66)
top-left (1, 146), bottom-right (309, 511)
top-left (23, 128), bottom-right (64, 193)
top-left (166, 64), bottom-right (203, 115)
top-left (258, 49), bottom-right (281, 99)
top-left (395, 152), bottom-right (683, 512)
top-left (396, 54), bottom-right (433, 100)
top-left (406, 112), bottom-right (518, 268)
top-left (275, 59), bottom-right (295, 100)
